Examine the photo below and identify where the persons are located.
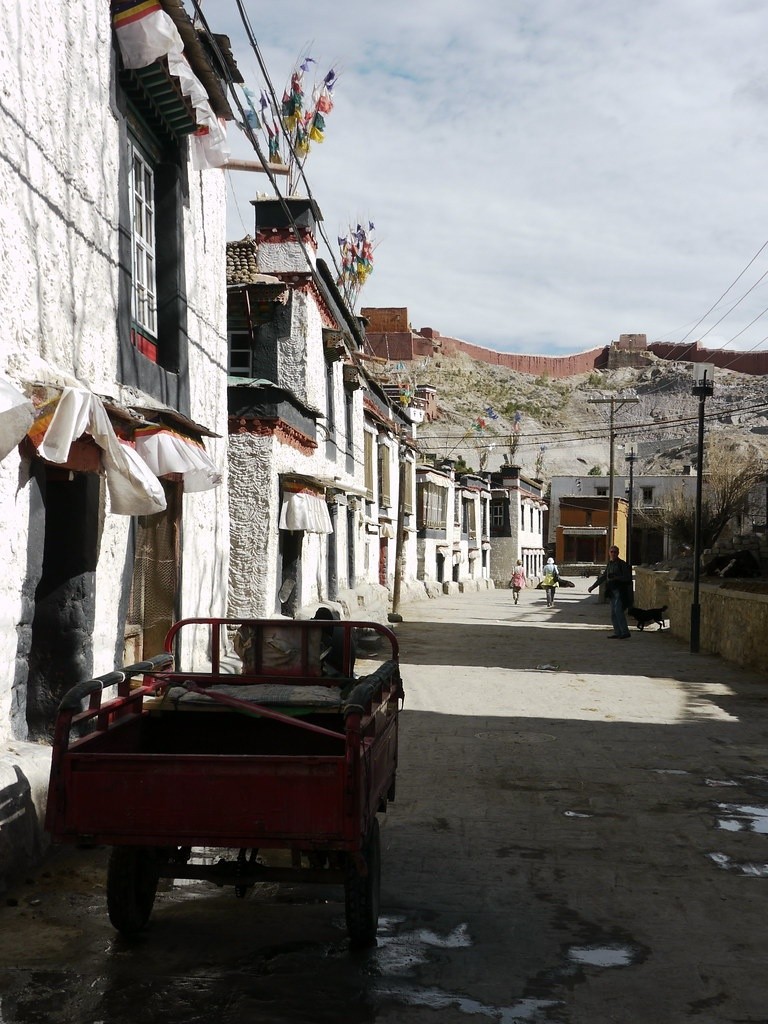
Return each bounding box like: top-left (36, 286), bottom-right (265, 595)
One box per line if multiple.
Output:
top-left (588, 546), bottom-right (631, 639)
top-left (541, 558), bottom-right (559, 607)
top-left (512, 559), bottom-right (528, 605)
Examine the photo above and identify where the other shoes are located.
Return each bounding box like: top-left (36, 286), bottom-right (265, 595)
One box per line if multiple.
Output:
top-left (608, 633), bottom-right (620, 639)
top-left (515, 598), bottom-right (518, 604)
top-left (547, 602), bottom-right (550, 607)
top-left (551, 602), bottom-right (554, 606)
top-left (620, 632), bottom-right (631, 639)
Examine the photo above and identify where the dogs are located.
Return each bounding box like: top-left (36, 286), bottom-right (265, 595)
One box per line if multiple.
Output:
top-left (581, 571), bottom-right (589, 578)
top-left (627, 604), bottom-right (669, 632)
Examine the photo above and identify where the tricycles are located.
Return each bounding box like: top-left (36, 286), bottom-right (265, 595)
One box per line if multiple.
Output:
top-left (50, 605), bottom-right (401, 952)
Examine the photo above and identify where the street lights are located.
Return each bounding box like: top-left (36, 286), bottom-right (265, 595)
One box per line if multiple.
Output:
top-left (689, 363), bottom-right (716, 652)
top-left (624, 440), bottom-right (639, 565)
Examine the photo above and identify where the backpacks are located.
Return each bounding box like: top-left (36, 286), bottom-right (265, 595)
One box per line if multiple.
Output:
top-left (509, 575), bottom-right (515, 589)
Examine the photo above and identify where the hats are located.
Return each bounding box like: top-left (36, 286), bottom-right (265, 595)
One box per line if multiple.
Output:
top-left (547, 558), bottom-right (554, 563)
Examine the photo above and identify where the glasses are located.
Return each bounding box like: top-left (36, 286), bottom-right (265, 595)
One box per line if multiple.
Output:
top-left (610, 550), bottom-right (619, 554)
